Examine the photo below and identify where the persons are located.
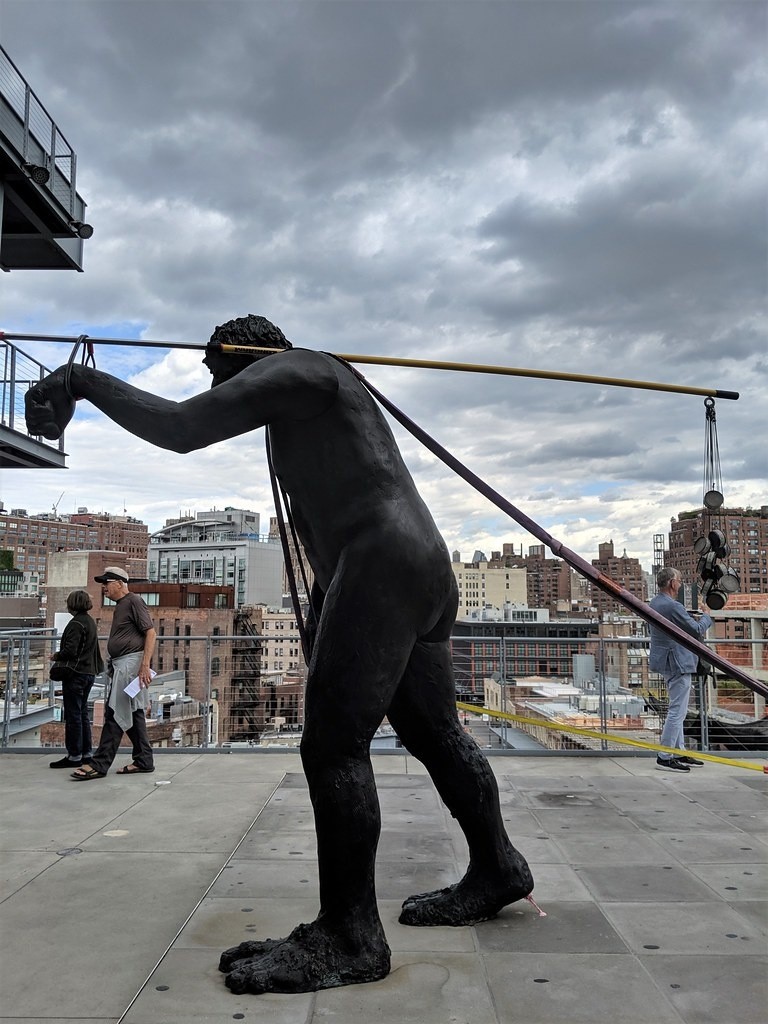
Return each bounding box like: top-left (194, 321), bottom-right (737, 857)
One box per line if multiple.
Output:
top-left (70, 567), bottom-right (157, 780)
top-left (24, 311), bottom-right (535, 994)
top-left (49, 590), bottom-right (105, 768)
top-left (646, 567), bottom-right (714, 773)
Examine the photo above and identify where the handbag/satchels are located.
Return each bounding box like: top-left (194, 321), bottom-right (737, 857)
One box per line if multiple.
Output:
top-left (49, 621), bottom-right (86, 680)
top-left (694, 634), bottom-right (717, 688)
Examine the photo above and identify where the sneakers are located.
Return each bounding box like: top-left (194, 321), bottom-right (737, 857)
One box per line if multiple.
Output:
top-left (656, 754), bottom-right (690, 773)
top-left (675, 755), bottom-right (705, 767)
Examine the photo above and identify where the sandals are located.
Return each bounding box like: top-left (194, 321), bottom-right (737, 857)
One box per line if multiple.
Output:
top-left (117, 763), bottom-right (155, 773)
top-left (71, 764), bottom-right (106, 779)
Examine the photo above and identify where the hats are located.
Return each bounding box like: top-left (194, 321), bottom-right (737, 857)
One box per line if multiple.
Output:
top-left (94, 566), bottom-right (129, 584)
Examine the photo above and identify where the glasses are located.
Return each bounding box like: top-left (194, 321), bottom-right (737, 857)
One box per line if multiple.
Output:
top-left (103, 579), bottom-right (115, 584)
top-left (678, 580), bottom-right (683, 584)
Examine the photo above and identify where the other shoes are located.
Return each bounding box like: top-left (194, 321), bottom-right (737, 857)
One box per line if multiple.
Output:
top-left (50, 757), bottom-right (82, 767)
top-left (80, 755), bottom-right (92, 763)
